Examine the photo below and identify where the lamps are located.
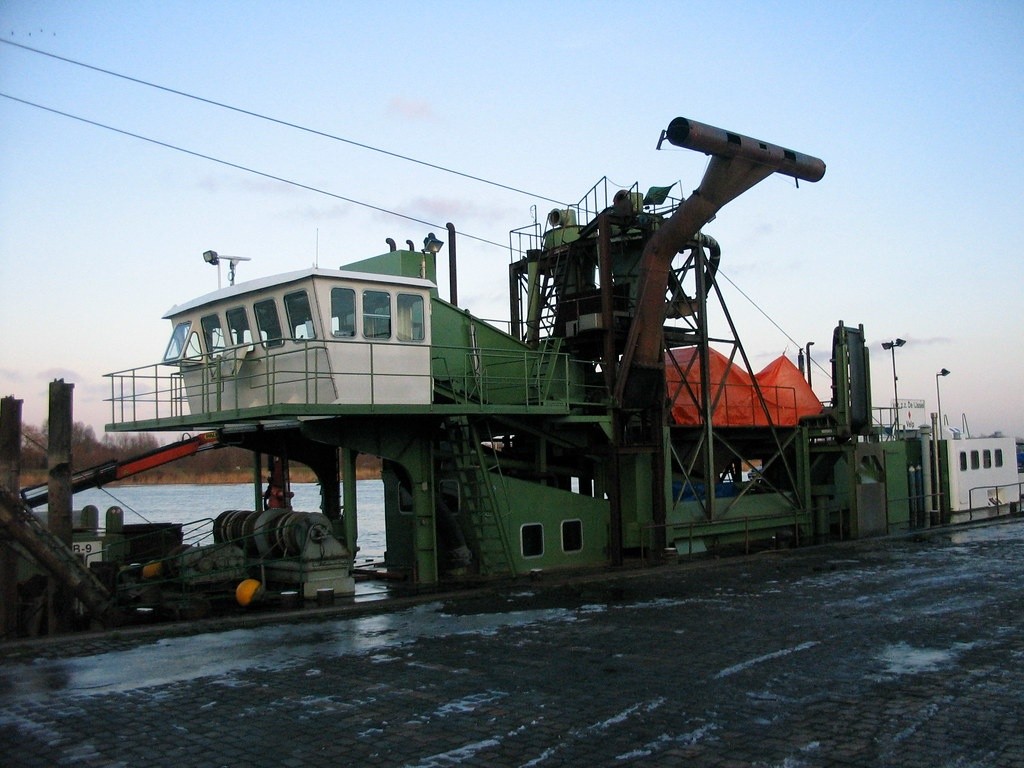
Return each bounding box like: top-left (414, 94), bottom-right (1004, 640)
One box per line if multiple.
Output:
top-left (203, 250), bottom-right (222, 290)
top-left (421, 237), bottom-right (444, 278)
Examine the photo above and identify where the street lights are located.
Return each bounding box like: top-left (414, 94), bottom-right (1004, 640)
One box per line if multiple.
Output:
top-left (936, 368), bottom-right (951, 440)
top-left (882, 337), bottom-right (905, 441)
